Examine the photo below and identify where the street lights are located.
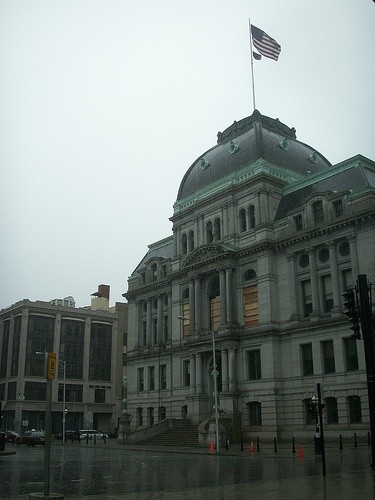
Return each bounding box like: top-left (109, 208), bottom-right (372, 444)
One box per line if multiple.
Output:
top-left (177, 316), bottom-right (220, 453)
top-left (35, 351), bottom-right (66, 444)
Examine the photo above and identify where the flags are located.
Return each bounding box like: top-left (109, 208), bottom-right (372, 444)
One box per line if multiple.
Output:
top-left (249, 18), bottom-right (282, 109)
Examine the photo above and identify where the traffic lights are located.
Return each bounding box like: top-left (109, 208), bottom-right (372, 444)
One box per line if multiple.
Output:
top-left (341, 290), bottom-right (356, 318)
top-left (348, 315), bottom-right (361, 339)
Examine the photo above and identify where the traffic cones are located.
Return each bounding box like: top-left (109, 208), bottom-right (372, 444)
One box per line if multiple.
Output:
top-left (297, 447), bottom-right (303, 457)
top-left (209, 440), bottom-right (215, 453)
top-left (249, 441), bottom-right (255, 452)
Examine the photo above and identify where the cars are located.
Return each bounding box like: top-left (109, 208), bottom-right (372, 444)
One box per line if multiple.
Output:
top-left (54, 431), bottom-right (78, 441)
top-left (5, 429), bottom-right (45, 446)
top-left (79, 430), bottom-right (109, 440)
